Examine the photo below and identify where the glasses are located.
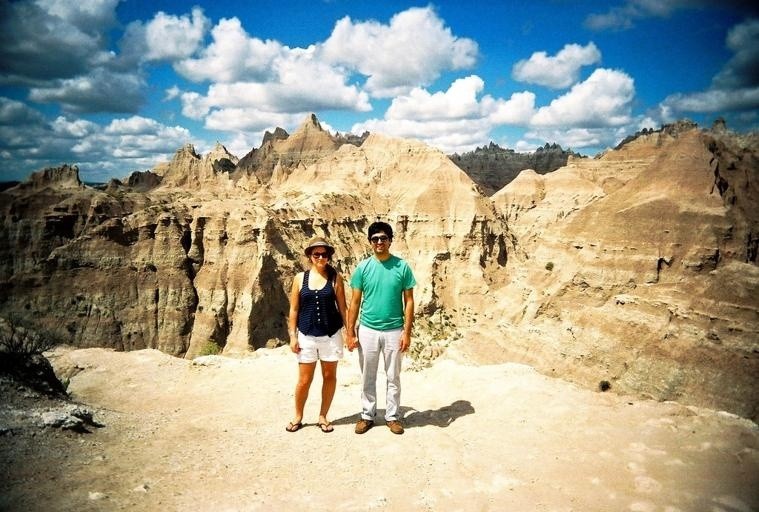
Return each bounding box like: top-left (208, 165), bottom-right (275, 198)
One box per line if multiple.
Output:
top-left (369, 235), bottom-right (390, 244)
top-left (308, 251), bottom-right (330, 258)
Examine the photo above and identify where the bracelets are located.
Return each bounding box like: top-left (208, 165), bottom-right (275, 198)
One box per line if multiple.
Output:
top-left (289, 331), bottom-right (297, 337)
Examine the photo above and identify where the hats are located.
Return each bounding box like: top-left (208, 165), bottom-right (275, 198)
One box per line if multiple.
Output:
top-left (303, 235), bottom-right (335, 260)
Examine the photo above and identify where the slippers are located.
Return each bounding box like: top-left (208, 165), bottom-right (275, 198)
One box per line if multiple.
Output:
top-left (317, 420), bottom-right (335, 432)
top-left (285, 419), bottom-right (302, 432)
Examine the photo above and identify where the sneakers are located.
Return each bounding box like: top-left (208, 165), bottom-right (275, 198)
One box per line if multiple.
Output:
top-left (385, 418), bottom-right (405, 434)
top-left (354, 418), bottom-right (375, 435)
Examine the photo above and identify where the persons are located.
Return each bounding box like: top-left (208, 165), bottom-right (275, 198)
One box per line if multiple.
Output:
top-left (346, 221), bottom-right (417, 435)
top-left (285, 237), bottom-right (360, 433)
